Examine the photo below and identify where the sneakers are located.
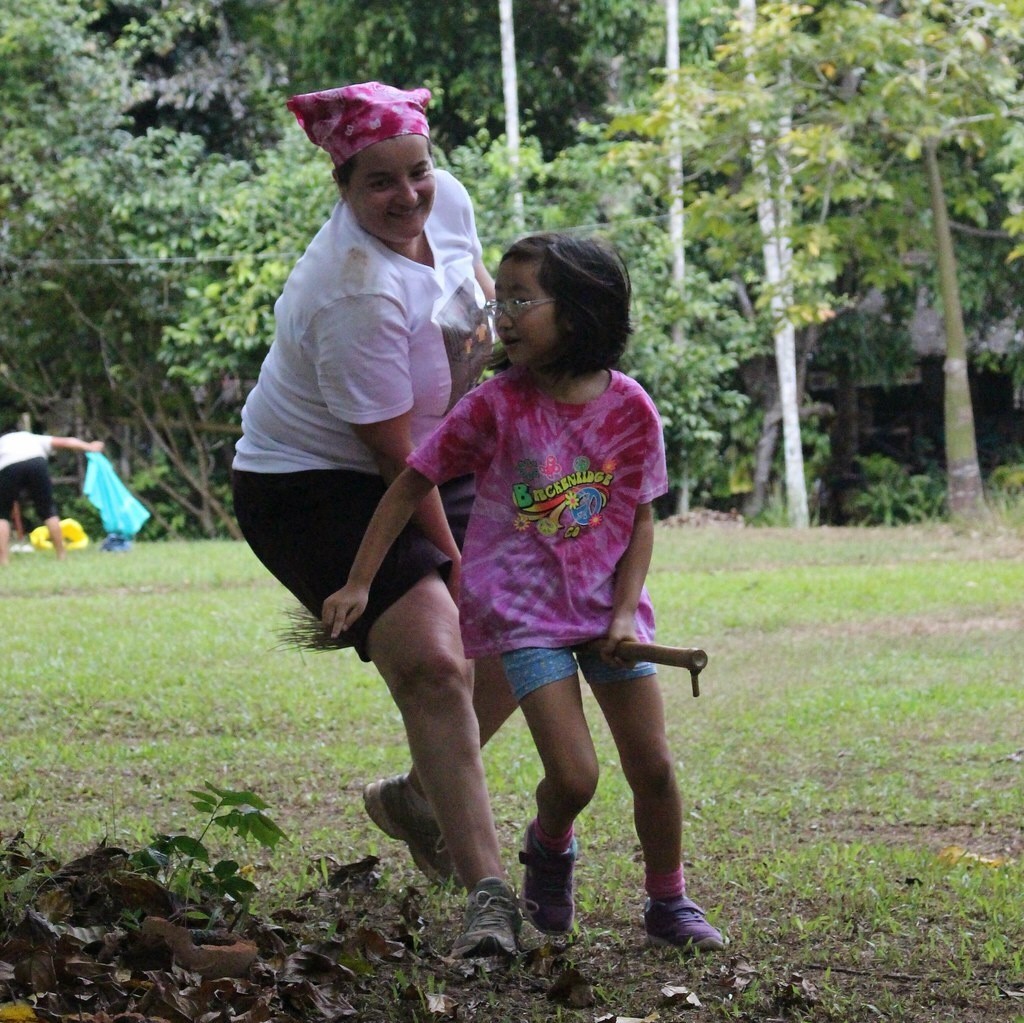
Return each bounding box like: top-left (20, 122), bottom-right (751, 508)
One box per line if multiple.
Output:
top-left (643, 896), bottom-right (726, 952)
top-left (451, 877), bottom-right (538, 960)
top-left (363, 774), bottom-right (463, 896)
top-left (519, 819), bottom-right (578, 937)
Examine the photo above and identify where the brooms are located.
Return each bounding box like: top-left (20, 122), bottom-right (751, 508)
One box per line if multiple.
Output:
top-left (243, 596), bottom-right (707, 696)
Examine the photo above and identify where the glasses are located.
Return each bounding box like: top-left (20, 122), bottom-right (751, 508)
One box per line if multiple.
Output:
top-left (484, 298), bottom-right (555, 319)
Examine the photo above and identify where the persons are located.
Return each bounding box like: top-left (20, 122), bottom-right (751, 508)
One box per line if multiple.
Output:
top-left (319, 230), bottom-right (734, 954)
top-left (227, 78), bottom-right (512, 958)
top-left (1, 429), bottom-right (106, 566)
